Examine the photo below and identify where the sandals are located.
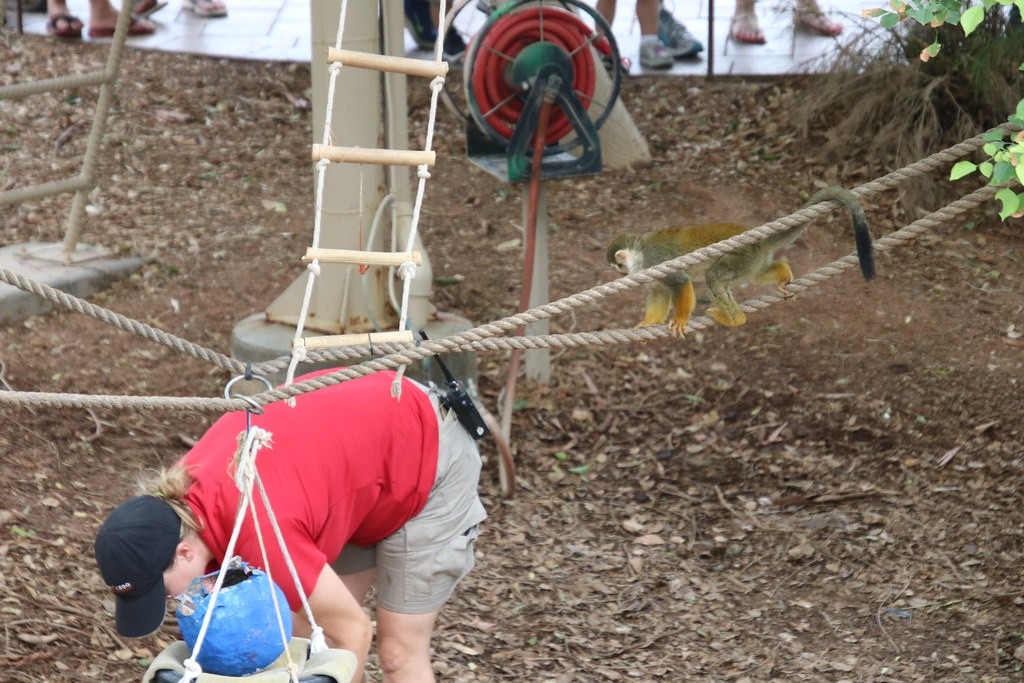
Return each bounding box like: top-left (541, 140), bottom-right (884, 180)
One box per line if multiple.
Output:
top-left (793, 6), bottom-right (841, 37)
top-left (731, 13), bottom-right (766, 46)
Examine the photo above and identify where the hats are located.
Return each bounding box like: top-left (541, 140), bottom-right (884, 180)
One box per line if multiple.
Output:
top-left (95, 493), bottom-right (181, 637)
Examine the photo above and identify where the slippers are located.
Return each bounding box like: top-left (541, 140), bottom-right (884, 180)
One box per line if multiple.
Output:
top-left (45, 15), bottom-right (84, 38)
top-left (88, 14), bottom-right (155, 37)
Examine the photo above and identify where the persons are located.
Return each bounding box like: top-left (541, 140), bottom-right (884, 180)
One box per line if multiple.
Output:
top-left (596, 1), bottom-right (674, 77)
top-left (658, 0), bottom-right (704, 56)
top-left (477, 0), bottom-right (516, 16)
top-left (404, 0), bottom-right (438, 51)
top-left (430, 0), bottom-right (466, 63)
top-left (95, 366), bottom-right (488, 683)
top-left (730, 0), bottom-right (844, 44)
top-left (131, 0), bottom-right (228, 17)
top-left (46, 0), bottom-right (157, 37)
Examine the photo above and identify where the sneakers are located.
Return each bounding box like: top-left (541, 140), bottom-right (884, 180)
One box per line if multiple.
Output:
top-left (639, 37), bottom-right (673, 69)
top-left (656, 9), bottom-right (703, 61)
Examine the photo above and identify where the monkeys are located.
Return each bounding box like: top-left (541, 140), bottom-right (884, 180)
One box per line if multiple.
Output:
top-left (606, 185), bottom-right (876, 338)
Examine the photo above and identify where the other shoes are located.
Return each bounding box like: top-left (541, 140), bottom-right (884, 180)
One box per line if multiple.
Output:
top-left (132, 0), bottom-right (167, 19)
top-left (183, 0), bottom-right (228, 16)
top-left (404, 0), bottom-right (436, 49)
top-left (440, 27), bottom-right (467, 63)
